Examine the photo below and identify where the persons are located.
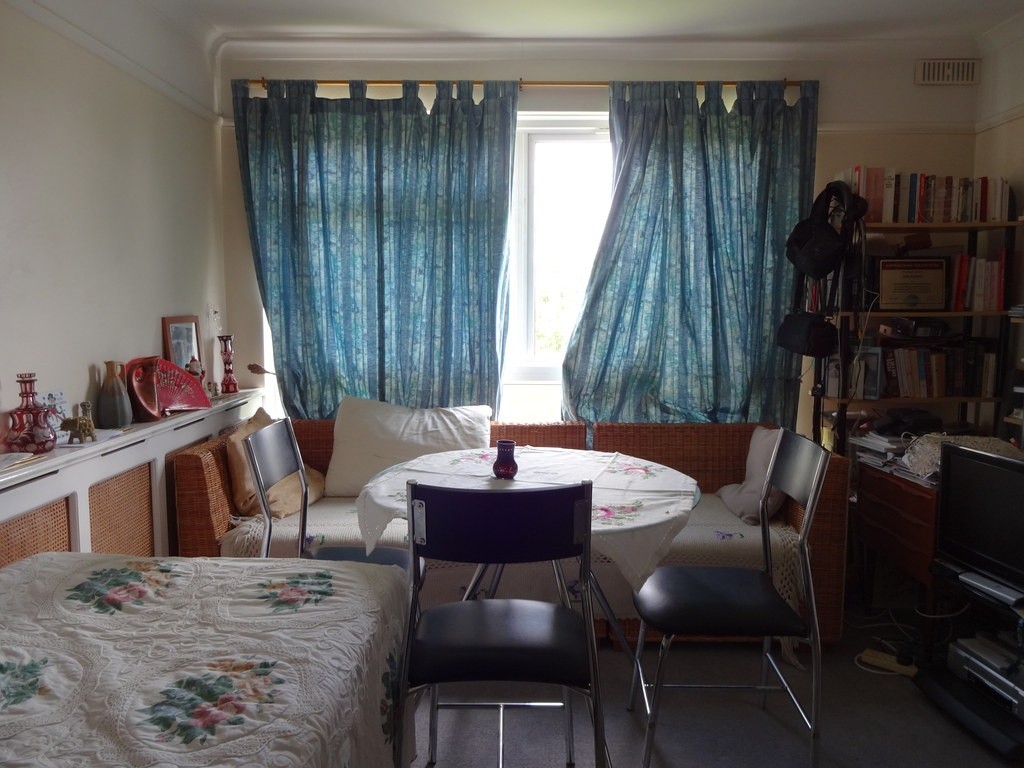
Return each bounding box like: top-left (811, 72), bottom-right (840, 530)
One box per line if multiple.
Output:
top-left (185, 356), bottom-right (206, 382)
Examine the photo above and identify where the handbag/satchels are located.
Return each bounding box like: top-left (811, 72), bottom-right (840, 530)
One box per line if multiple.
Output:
top-left (786, 180), bottom-right (870, 279)
top-left (778, 312), bottom-right (837, 357)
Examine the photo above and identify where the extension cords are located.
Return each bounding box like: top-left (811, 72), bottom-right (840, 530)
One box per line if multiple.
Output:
top-left (861, 649), bottom-right (919, 677)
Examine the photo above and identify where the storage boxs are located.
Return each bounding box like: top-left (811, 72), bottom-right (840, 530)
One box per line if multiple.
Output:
top-left (823, 407), bottom-right (978, 456)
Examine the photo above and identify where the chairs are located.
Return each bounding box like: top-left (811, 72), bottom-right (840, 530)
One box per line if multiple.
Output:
top-left (628, 428), bottom-right (832, 768)
top-left (244, 415), bottom-right (426, 723)
top-left (394, 481), bottom-right (605, 768)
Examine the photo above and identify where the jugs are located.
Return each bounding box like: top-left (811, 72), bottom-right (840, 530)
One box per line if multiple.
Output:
top-left (97, 361), bottom-right (132, 428)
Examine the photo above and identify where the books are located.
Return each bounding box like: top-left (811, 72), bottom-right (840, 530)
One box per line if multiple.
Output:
top-left (1012, 357), bottom-right (1024, 420)
top-left (829, 333), bottom-right (996, 397)
top-left (1008, 304), bottom-right (1024, 316)
top-left (0, 453), bottom-right (47, 472)
top-left (842, 248), bottom-right (1006, 311)
top-left (958, 631), bottom-right (1024, 672)
top-left (852, 163), bottom-right (1010, 224)
top-left (847, 431), bottom-right (938, 488)
top-left (1017, 215), bottom-right (1024, 222)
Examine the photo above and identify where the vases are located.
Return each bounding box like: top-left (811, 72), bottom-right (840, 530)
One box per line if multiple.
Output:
top-left (95, 361), bottom-right (132, 428)
top-left (5, 373), bottom-right (57, 454)
top-left (493, 440), bottom-right (518, 480)
top-left (217, 334), bottom-right (238, 393)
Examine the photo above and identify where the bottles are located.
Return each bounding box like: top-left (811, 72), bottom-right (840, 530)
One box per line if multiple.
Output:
top-left (493, 439), bottom-right (518, 479)
top-left (3, 373), bottom-right (58, 454)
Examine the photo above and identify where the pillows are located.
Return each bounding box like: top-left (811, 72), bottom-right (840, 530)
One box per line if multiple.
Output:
top-left (324, 396), bottom-right (494, 496)
top-left (226, 407), bottom-right (326, 520)
top-left (716, 424), bottom-right (787, 527)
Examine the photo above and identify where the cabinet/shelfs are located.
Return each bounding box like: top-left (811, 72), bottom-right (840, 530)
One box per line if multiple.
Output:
top-left (812, 185), bottom-right (1024, 638)
top-left (911, 561), bottom-right (1024, 767)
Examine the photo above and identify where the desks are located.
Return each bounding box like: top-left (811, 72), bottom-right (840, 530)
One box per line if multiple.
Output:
top-left (355, 446), bottom-right (701, 684)
top-left (0, 552), bottom-right (417, 768)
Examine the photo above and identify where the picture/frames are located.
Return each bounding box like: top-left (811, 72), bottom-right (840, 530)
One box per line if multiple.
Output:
top-left (162, 314), bottom-right (204, 375)
top-left (824, 347), bottom-right (881, 402)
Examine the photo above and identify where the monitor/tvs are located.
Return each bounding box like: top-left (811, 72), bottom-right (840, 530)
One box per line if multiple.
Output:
top-left (935, 441), bottom-right (1024, 611)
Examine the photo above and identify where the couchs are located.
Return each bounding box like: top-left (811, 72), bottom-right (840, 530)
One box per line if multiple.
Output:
top-left (173, 418), bottom-right (852, 671)
